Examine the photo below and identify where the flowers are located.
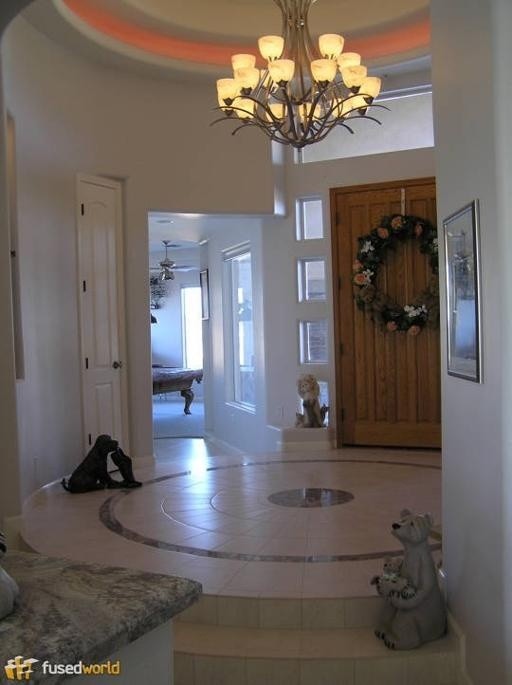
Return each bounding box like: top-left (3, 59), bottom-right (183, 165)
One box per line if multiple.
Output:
top-left (350, 213), bottom-right (439, 335)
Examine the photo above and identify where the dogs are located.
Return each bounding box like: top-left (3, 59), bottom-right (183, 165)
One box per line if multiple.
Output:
top-left (62, 434), bottom-right (125, 494)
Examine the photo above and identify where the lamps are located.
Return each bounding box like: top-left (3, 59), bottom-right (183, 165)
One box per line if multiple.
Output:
top-left (207, 0), bottom-right (391, 152)
top-left (158, 241), bottom-right (173, 281)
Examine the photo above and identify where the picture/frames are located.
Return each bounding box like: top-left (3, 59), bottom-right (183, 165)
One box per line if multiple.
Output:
top-left (443, 199), bottom-right (482, 389)
top-left (199, 269), bottom-right (210, 321)
